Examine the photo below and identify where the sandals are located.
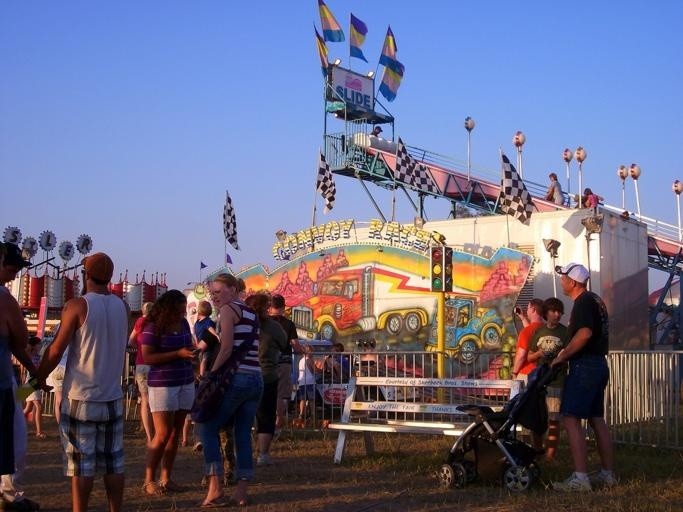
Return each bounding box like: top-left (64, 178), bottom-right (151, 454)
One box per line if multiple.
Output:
top-left (141, 477), bottom-right (177, 497)
top-left (35, 431), bottom-right (48, 441)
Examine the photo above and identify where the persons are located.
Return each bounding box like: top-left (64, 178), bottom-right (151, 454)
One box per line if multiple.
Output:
top-left (526, 298), bottom-right (569, 468)
top-left (548, 262), bottom-right (614, 494)
top-left (371, 126), bottom-right (383, 136)
top-left (510, 298), bottom-right (546, 400)
top-left (546, 173), bottom-right (566, 205)
top-left (584, 187), bottom-right (604, 208)
top-left (126, 274), bottom-right (306, 507)
top-left (0, 243), bottom-right (130, 512)
top-left (296, 342), bottom-right (351, 419)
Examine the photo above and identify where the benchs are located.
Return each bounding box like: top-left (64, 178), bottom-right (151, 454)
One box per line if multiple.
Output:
top-left (323, 377), bottom-right (524, 465)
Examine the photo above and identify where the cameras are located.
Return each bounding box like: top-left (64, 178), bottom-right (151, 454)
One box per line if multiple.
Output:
top-left (514, 307), bottom-right (520, 314)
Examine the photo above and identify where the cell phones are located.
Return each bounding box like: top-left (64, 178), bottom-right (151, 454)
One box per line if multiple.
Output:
top-left (193, 348), bottom-right (201, 352)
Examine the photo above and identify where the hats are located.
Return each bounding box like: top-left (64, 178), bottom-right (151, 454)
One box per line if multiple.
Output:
top-left (554, 262), bottom-right (591, 284)
top-left (0, 241), bottom-right (33, 266)
top-left (81, 253), bottom-right (114, 282)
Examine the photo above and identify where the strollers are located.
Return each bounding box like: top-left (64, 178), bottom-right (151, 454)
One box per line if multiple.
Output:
top-left (433, 362), bottom-right (562, 495)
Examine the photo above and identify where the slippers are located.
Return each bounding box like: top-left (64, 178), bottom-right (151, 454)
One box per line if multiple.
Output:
top-left (230, 494), bottom-right (248, 507)
top-left (199, 499), bottom-right (229, 508)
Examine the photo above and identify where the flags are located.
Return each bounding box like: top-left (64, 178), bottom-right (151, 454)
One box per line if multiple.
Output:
top-left (380, 60), bottom-right (405, 101)
top-left (318, 1), bottom-right (345, 42)
top-left (314, 25), bottom-right (329, 77)
top-left (223, 195), bottom-right (240, 251)
top-left (380, 28), bottom-right (398, 68)
top-left (315, 153), bottom-right (337, 215)
top-left (499, 153), bottom-right (532, 227)
top-left (350, 12), bottom-right (368, 63)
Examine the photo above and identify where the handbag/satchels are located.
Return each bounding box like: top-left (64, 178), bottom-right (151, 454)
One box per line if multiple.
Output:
top-left (189, 343), bottom-right (247, 423)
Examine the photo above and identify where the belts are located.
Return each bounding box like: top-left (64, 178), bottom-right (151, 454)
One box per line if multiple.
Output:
top-left (236, 368), bottom-right (257, 374)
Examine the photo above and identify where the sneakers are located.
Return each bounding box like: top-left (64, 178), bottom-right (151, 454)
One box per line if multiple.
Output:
top-left (588, 468), bottom-right (615, 489)
top-left (552, 472), bottom-right (593, 492)
top-left (193, 442), bottom-right (202, 454)
top-left (2, 498), bottom-right (40, 512)
top-left (256, 452), bottom-right (273, 466)
top-left (298, 414), bottom-right (304, 419)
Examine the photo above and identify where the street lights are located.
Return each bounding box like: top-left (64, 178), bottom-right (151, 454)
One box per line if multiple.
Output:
top-left (617, 163), bottom-right (629, 210)
top-left (511, 128), bottom-right (526, 179)
top-left (627, 162), bottom-right (643, 219)
top-left (572, 145), bottom-right (586, 208)
top-left (463, 114), bottom-right (474, 183)
top-left (669, 179), bottom-right (682, 243)
top-left (539, 234), bottom-right (560, 298)
top-left (559, 147), bottom-right (572, 195)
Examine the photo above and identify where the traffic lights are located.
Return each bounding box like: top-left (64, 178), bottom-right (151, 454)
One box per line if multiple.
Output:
top-left (430, 246), bottom-right (444, 293)
top-left (443, 246), bottom-right (453, 293)
